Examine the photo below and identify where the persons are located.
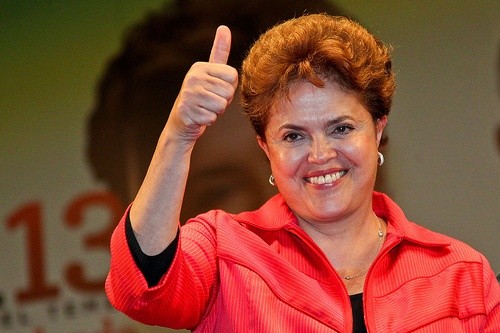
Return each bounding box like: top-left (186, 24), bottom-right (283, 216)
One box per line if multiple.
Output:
top-left (105, 14), bottom-right (500, 333)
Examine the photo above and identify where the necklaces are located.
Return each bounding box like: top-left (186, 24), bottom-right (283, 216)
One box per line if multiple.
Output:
top-left (340, 212), bottom-right (383, 280)
